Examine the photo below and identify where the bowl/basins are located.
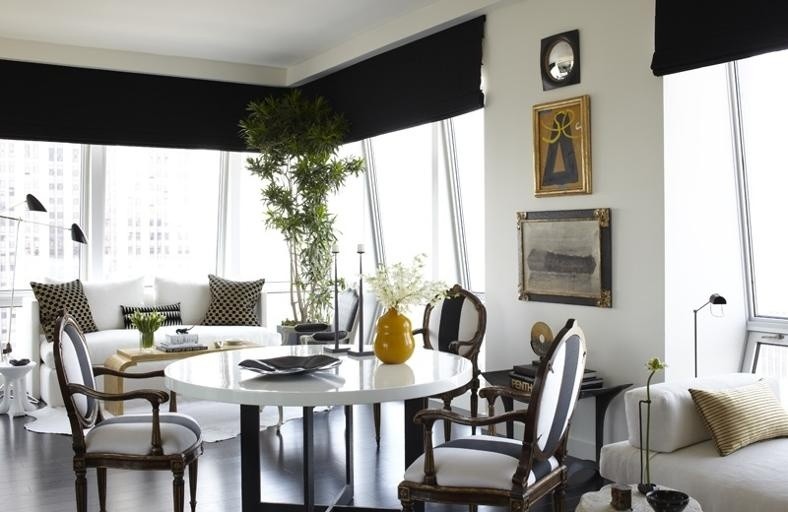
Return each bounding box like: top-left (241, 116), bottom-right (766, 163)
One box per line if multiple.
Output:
top-left (646, 490), bottom-right (690, 512)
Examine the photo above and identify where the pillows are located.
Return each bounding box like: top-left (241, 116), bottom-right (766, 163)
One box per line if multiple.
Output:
top-left (201, 273), bottom-right (264, 327)
top-left (44, 275), bottom-right (144, 329)
top-left (155, 277), bottom-right (210, 324)
top-left (688, 378), bottom-right (788, 458)
top-left (625, 372), bottom-right (780, 454)
top-left (29, 279), bottom-right (98, 342)
top-left (120, 302), bottom-right (182, 328)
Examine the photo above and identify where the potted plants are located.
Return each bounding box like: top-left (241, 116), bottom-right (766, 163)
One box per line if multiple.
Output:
top-left (239, 95), bottom-right (349, 345)
top-left (130, 308), bottom-right (166, 347)
top-left (638, 357), bottom-right (664, 494)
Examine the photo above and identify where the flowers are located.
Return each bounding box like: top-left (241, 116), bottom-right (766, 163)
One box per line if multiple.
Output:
top-left (367, 253), bottom-right (455, 304)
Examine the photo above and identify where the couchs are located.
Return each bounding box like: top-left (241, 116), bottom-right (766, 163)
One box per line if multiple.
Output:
top-left (16, 290), bottom-right (275, 411)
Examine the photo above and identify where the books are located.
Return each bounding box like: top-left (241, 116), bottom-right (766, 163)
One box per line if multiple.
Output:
top-left (155, 342), bottom-right (209, 352)
top-left (508, 363), bottom-right (603, 393)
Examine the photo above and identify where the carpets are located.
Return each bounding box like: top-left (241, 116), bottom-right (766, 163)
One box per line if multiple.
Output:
top-left (23, 404), bottom-right (333, 442)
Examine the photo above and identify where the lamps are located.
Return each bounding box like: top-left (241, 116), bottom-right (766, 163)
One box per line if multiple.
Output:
top-left (693, 293), bottom-right (727, 377)
top-left (0, 194), bottom-right (89, 360)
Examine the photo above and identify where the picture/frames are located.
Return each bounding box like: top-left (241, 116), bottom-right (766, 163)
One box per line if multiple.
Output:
top-left (532, 95), bottom-right (591, 198)
top-left (539, 29), bottom-right (580, 91)
top-left (516, 207), bottom-right (612, 308)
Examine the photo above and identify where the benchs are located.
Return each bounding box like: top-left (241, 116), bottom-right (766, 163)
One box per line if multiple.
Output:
top-left (599, 435), bottom-right (788, 512)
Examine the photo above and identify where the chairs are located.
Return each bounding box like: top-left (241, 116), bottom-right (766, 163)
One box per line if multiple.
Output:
top-left (51, 307), bottom-right (204, 512)
top-left (398, 318), bottom-right (588, 512)
top-left (412, 284), bottom-right (487, 442)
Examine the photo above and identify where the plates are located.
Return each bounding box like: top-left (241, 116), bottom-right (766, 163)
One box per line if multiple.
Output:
top-left (9, 358), bottom-right (30, 366)
top-left (237, 355), bottom-right (339, 377)
top-left (225, 340), bottom-right (243, 345)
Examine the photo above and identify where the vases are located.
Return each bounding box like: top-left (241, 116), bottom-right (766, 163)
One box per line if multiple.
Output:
top-left (374, 308), bottom-right (414, 364)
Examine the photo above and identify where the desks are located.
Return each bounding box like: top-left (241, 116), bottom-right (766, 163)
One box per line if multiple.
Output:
top-left (0, 358), bottom-right (36, 420)
top-left (481, 362), bottom-right (633, 464)
top-left (164, 346), bottom-right (473, 512)
